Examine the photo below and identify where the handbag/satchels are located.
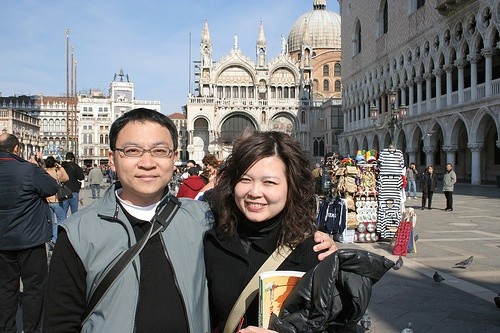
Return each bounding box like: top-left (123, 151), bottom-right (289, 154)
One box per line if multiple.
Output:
top-left (55, 167), bottom-right (73, 201)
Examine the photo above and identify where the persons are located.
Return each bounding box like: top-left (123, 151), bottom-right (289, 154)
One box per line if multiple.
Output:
top-left (28, 151), bottom-right (85, 257)
top-left (101, 164), bottom-right (117, 185)
top-left (443, 163), bottom-right (456, 212)
top-left (202, 131), bottom-right (396, 333)
top-left (39, 108), bottom-right (339, 333)
top-left (171, 153), bottom-right (224, 201)
top-left (420, 166), bottom-right (437, 210)
top-left (312, 163), bottom-right (323, 177)
top-left (88, 163), bottom-right (103, 199)
top-left (405, 163), bottom-right (418, 199)
top-left (0, 132), bottom-right (58, 333)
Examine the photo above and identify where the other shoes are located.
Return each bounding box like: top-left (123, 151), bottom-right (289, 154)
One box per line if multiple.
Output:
top-left (421, 207), bottom-right (424, 210)
top-left (445, 208), bottom-right (453, 211)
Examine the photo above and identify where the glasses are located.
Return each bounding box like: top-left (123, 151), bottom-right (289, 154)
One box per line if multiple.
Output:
top-left (115, 146), bottom-right (176, 158)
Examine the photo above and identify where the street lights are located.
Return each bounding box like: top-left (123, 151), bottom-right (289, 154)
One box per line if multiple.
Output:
top-left (369, 87), bottom-right (408, 148)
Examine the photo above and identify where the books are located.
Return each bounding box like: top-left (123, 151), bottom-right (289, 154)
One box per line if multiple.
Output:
top-left (258, 271), bottom-right (305, 328)
